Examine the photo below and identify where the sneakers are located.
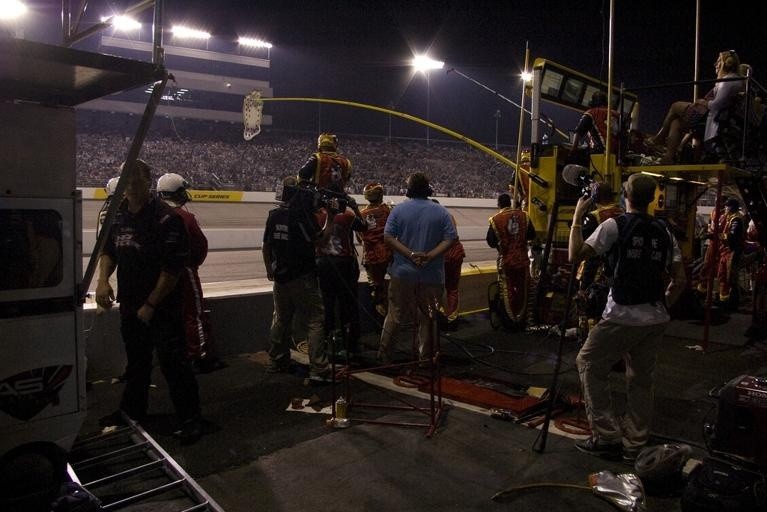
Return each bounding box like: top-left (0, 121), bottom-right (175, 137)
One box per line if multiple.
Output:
top-left (99, 408), bottom-right (147, 434)
top-left (267, 360), bottom-right (296, 375)
top-left (575, 435), bottom-right (622, 456)
top-left (308, 368), bottom-right (346, 384)
top-left (623, 449), bottom-right (639, 460)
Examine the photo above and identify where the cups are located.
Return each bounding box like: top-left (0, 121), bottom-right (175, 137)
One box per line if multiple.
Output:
top-left (292, 397), bottom-right (304, 409)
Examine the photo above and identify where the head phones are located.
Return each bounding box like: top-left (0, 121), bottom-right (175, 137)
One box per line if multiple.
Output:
top-left (724, 50), bottom-right (737, 71)
top-left (163, 187), bottom-right (194, 204)
top-left (586, 98), bottom-right (594, 109)
top-left (405, 172), bottom-right (434, 198)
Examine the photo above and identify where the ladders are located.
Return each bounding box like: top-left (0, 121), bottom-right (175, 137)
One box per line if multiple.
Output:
top-left (66, 410), bottom-right (225, 512)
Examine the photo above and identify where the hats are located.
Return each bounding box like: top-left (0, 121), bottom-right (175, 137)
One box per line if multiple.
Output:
top-left (622, 172), bottom-right (657, 200)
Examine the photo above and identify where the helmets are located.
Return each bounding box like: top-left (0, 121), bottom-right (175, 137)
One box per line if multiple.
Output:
top-left (723, 199), bottom-right (740, 211)
top-left (106, 176), bottom-right (122, 197)
top-left (318, 132), bottom-right (339, 152)
top-left (156, 172), bottom-right (192, 207)
top-left (363, 182), bottom-right (383, 204)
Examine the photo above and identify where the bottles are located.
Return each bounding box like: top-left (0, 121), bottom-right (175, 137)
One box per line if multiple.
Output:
top-left (326, 418), bottom-right (350, 428)
top-left (335, 396), bottom-right (346, 419)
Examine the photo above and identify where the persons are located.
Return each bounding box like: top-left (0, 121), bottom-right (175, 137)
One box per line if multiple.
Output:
top-left (568, 172), bottom-right (687, 462)
top-left (262, 132), bottom-right (466, 390)
top-left (484, 48), bottom-right (767, 334)
top-left (96, 159), bottom-right (208, 444)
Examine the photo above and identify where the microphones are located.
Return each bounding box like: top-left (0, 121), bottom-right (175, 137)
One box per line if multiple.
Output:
top-left (562, 163), bottom-right (594, 187)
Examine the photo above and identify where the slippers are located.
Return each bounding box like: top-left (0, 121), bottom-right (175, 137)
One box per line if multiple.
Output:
top-left (642, 137), bottom-right (666, 154)
